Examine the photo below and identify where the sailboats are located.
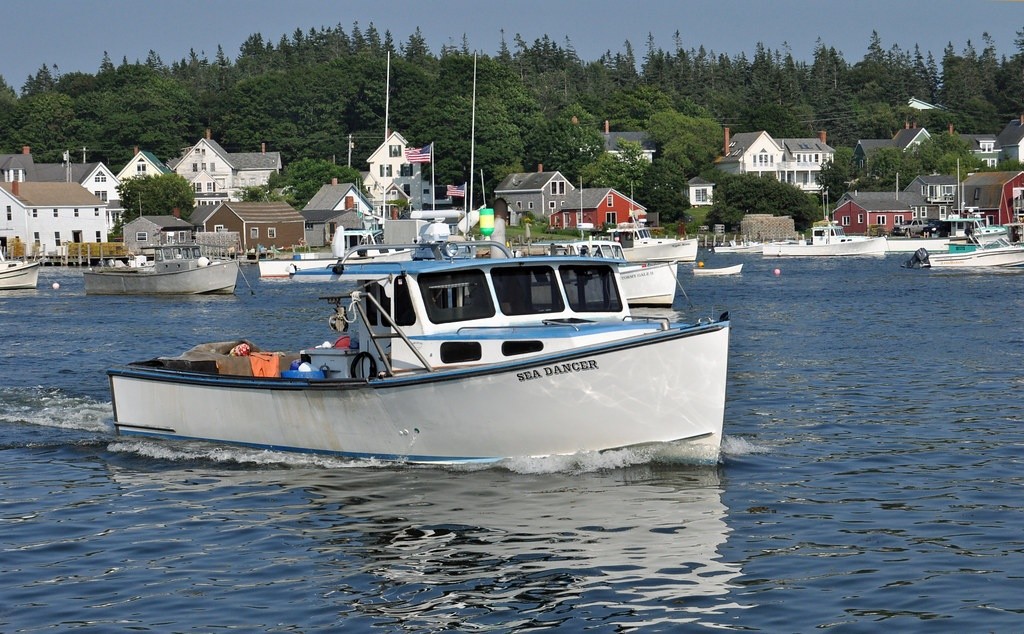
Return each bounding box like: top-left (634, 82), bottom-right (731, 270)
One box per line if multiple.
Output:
top-left (256, 50), bottom-right (517, 282)
top-left (762, 186), bottom-right (887, 256)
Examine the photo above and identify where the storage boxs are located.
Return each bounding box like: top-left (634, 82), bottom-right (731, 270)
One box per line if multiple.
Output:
top-left (300, 348), bottom-right (359, 379)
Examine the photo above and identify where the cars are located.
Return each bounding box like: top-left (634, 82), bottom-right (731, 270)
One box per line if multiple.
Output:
top-left (921, 220), bottom-right (951, 237)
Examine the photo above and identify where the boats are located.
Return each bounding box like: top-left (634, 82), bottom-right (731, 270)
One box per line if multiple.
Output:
top-left (713, 240), bottom-right (768, 253)
top-left (693, 263), bottom-right (744, 276)
top-left (511, 176), bottom-right (589, 256)
top-left (570, 181), bottom-right (699, 263)
top-left (410, 209), bottom-right (678, 307)
top-left (82, 244), bottom-right (241, 296)
top-left (107, 240), bottom-right (730, 472)
top-left (901, 182), bottom-right (1024, 269)
top-left (0, 241), bottom-right (46, 288)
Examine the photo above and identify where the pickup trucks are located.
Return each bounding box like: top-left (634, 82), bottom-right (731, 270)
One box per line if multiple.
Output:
top-left (893, 219), bottom-right (928, 236)
